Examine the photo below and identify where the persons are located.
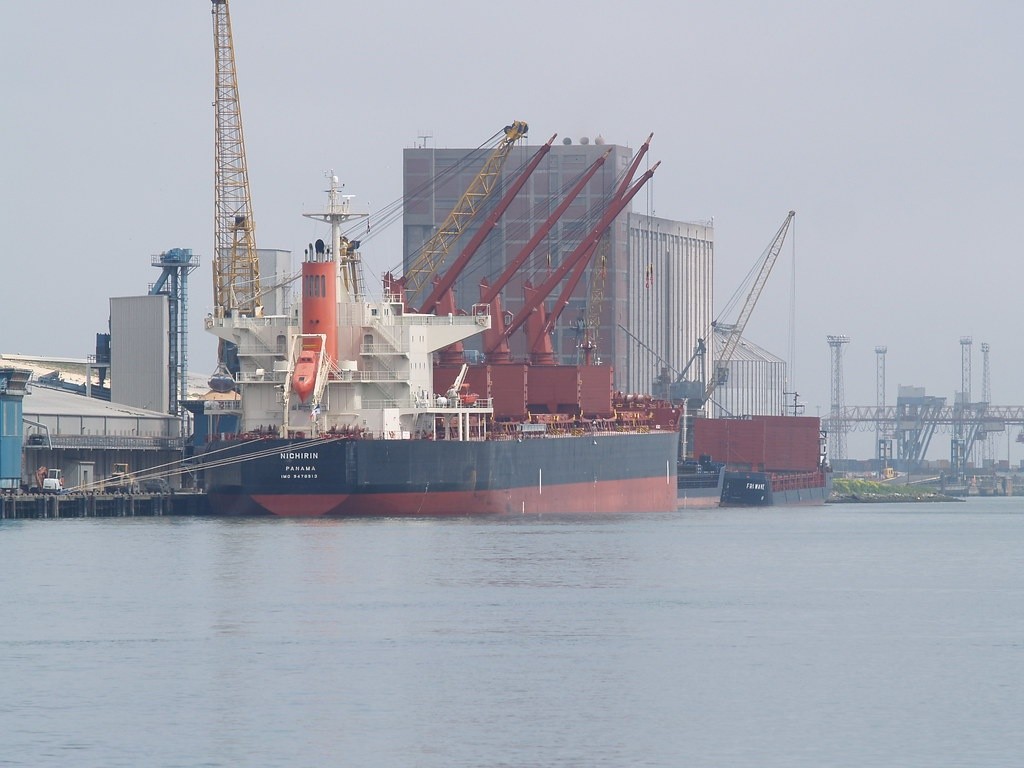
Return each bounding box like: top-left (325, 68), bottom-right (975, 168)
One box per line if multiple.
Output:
top-left (60, 476), bottom-right (64, 486)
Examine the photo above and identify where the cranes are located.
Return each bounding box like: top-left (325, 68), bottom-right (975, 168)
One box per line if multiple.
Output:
top-left (198, 1), bottom-right (797, 443)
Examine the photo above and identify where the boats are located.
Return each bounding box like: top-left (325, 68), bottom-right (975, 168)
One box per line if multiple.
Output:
top-left (290, 349), bottom-right (319, 405)
top-left (459, 392), bottom-right (479, 406)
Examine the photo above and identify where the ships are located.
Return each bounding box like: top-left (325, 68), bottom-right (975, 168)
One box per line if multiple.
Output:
top-left (195, 168), bottom-right (829, 525)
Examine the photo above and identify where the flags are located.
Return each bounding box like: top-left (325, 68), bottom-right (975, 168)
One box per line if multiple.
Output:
top-left (310, 404), bottom-right (320, 419)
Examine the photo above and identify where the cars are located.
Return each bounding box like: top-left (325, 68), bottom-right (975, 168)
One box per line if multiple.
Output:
top-left (144, 478), bottom-right (170, 495)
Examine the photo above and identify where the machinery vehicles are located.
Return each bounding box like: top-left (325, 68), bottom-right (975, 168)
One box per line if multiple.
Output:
top-left (41, 468), bottom-right (65, 494)
top-left (105, 463), bottom-right (141, 496)
